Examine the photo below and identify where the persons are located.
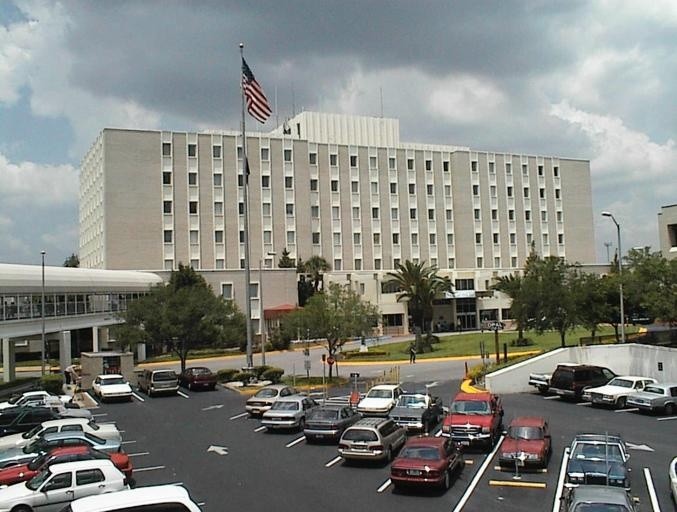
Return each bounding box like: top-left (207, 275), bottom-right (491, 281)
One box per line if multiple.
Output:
top-left (409, 341), bottom-right (417, 364)
top-left (361, 336), bottom-right (367, 346)
top-left (1, 297), bottom-right (55, 321)
top-left (63, 362), bottom-right (80, 390)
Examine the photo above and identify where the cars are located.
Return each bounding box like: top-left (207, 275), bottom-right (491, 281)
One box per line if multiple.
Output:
top-left (245, 385), bottom-right (308, 417)
top-left (529, 372), bottom-right (552, 393)
top-left (0, 431), bottom-right (125, 468)
top-left (0, 391), bottom-right (95, 437)
top-left (338, 418), bottom-right (406, 462)
top-left (566, 434), bottom-right (632, 492)
top-left (261, 397), bottom-right (321, 431)
top-left (0, 418), bottom-right (123, 453)
top-left (0, 446), bottom-right (133, 485)
top-left (303, 406), bottom-right (361, 441)
top-left (563, 485), bottom-right (640, 511)
top-left (389, 437), bottom-right (465, 490)
top-left (495, 416), bottom-right (552, 468)
top-left (91, 374), bottom-right (133, 402)
top-left (357, 385), bottom-right (403, 416)
top-left (668, 456), bottom-right (677, 506)
top-left (582, 376), bottom-right (659, 409)
top-left (626, 384), bottom-right (677, 416)
top-left (0, 459), bottom-right (130, 512)
top-left (178, 367), bottom-right (218, 390)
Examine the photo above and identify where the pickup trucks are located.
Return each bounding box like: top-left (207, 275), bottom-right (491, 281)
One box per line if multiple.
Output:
top-left (442, 393), bottom-right (504, 452)
top-left (389, 393), bottom-right (443, 433)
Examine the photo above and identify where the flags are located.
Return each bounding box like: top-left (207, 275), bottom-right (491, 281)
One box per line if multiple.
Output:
top-left (241, 57), bottom-right (273, 124)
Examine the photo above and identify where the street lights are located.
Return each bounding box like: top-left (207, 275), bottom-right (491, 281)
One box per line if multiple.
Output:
top-left (258, 251), bottom-right (277, 366)
top-left (602, 211), bottom-right (626, 343)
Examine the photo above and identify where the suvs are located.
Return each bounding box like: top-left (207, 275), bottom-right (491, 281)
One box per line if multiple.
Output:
top-left (139, 369), bottom-right (180, 396)
top-left (548, 364), bottom-right (618, 402)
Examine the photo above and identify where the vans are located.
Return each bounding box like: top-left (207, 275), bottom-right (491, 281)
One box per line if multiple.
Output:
top-left (58, 485), bottom-right (203, 512)
